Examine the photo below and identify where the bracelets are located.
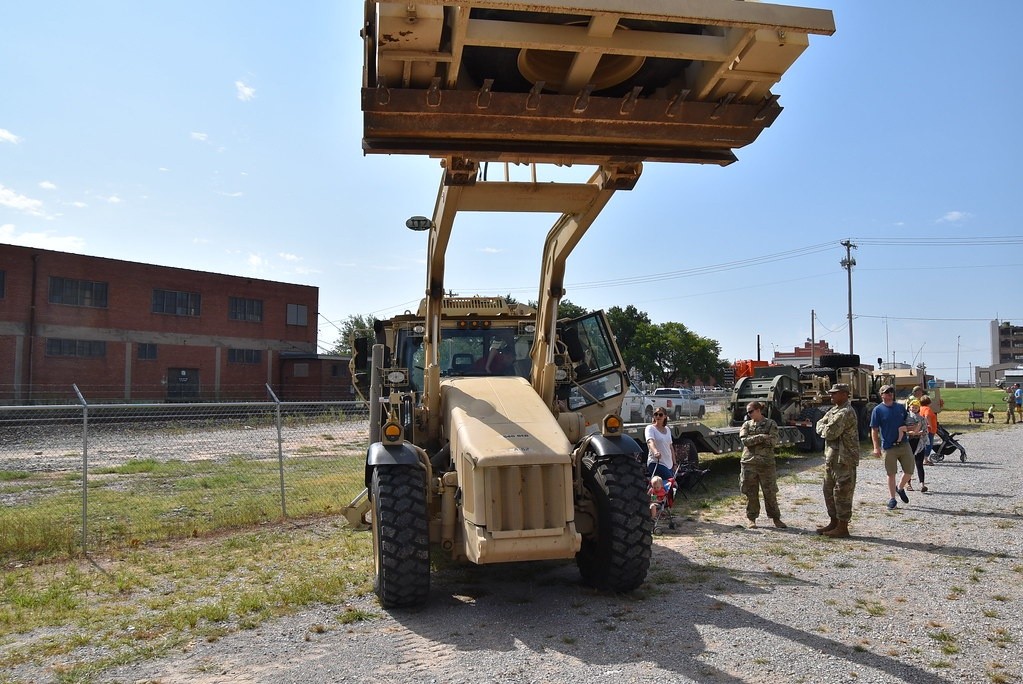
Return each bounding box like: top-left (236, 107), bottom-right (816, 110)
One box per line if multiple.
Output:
top-left (674, 464), bottom-right (678, 465)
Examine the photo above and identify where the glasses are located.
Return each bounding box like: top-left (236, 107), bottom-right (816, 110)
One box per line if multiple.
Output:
top-left (654, 412), bottom-right (666, 417)
top-left (882, 389), bottom-right (894, 394)
top-left (746, 408), bottom-right (758, 415)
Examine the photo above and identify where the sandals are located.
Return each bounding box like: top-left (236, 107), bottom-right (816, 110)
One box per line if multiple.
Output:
top-left (920, 486), bottom-right (928, 492)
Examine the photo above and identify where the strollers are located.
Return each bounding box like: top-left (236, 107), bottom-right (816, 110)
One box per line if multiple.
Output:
top-left (929, 418), bottom-right (967, 463)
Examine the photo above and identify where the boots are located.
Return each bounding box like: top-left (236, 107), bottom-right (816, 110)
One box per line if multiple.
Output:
top-left (817, 517), bottom-right (849, 538)
top-left (773, 518), bottom-right (786, 528)
top-left (747, 518), bottom-right (756, 528)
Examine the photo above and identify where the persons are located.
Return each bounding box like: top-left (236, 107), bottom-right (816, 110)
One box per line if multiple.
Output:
top-left (643, 407), bottom-right (678, 523)
top-left (869, 385), bottom-right (944, 510)
top-left (986, 403), bottom-right (996, 423)
top-left (1002, 383), bottom-right (1023, 425)
top-left (472, 346), bottom-right (516, 376)
top-left (815, 383), bottom-right (861, 539)
top-left (739, 401), bottom-right (786, 529)
top-left (928, 377), bottom-right (935, 392)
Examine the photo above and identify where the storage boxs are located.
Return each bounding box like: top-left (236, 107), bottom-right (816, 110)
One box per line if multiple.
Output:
top-left (970, 411), bottom-right (984, 417)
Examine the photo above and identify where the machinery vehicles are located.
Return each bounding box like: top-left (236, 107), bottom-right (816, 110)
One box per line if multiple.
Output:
top-left (358, 1), bottom-right (841, 611)
top-left (611, 353), bottom-right (927, 475)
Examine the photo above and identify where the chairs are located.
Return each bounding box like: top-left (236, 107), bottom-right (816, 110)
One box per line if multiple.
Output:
top-left (452, 353), bottom-right (475, 369)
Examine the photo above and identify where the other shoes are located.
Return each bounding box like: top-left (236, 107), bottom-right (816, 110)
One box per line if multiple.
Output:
top-left (923, 461), bottom-right (934, 466)
top-left (907, 484), bottom-right (913, 491)
top-left (651, 517), bottom-right (659, 524)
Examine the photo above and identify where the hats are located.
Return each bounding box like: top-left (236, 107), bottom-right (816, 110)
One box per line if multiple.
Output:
top-left (912, 386), bottom-right (921, 393)
top-left (879, 385), bottom-right (895, 394)
top-left (909, 400), bottom-right (920, 407)
top-left (828, 384), bottom-right (849, 392)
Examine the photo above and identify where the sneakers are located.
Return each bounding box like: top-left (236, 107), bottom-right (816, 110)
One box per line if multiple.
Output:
top-left (895, 486), bottom-right (909, 504)
top-left (887, 498), bottom-right (897, 510)
